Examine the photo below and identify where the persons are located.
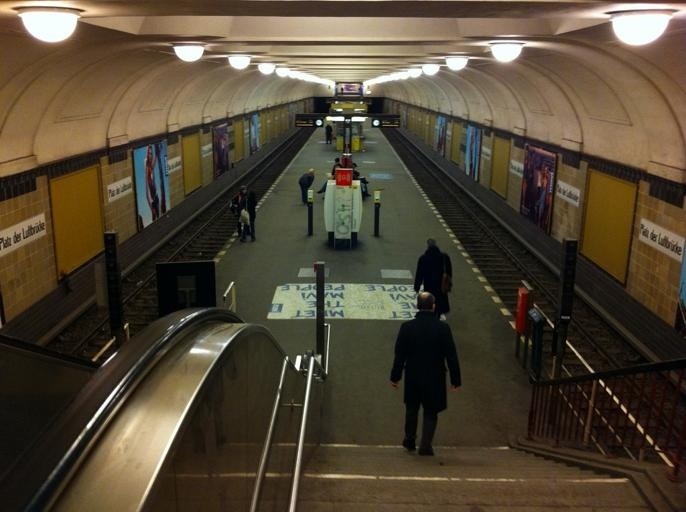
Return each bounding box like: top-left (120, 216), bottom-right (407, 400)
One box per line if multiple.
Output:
top-left (390, 291), bottom-right (462, 457)
top-left (317, 158), bottom-right (372, 201)
top-left (298, 168), bottom-right (315, 204)
top-left (530, 159), bottom-right (550, 229)
top-left (229, 184), bottom-right (256, 242)
top-left (144, 144), bottom-right (160, 222)
top-left (325, 123), bottom-right (333, 144)
top-left (413, 239), bottom-right (453, 321)
top-left (217, 132), bottom-right (229, 174)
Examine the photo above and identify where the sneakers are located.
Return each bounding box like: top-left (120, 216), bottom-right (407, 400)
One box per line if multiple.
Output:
top-left (403, 439), bottom-right (416, 451)
top-left (419, 448), bottom-right (434, 456)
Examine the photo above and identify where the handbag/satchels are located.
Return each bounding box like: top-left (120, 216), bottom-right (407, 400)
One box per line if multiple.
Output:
top-left (239, 210), bottom-right (249, 224)
top-left (442, 274), bottom-right (450, 292)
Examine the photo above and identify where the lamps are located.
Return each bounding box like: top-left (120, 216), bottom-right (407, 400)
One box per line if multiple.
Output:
top-left (359, 57), bottom-right (470, 88)
top-left (170, 41), bottom-right (338, 86)
top-left (484, 43), bottom-right (527, 64)
top-left (11, 9), bottom-right (85, 47)
top-left (607, 9), bottom-right (682, 49)
